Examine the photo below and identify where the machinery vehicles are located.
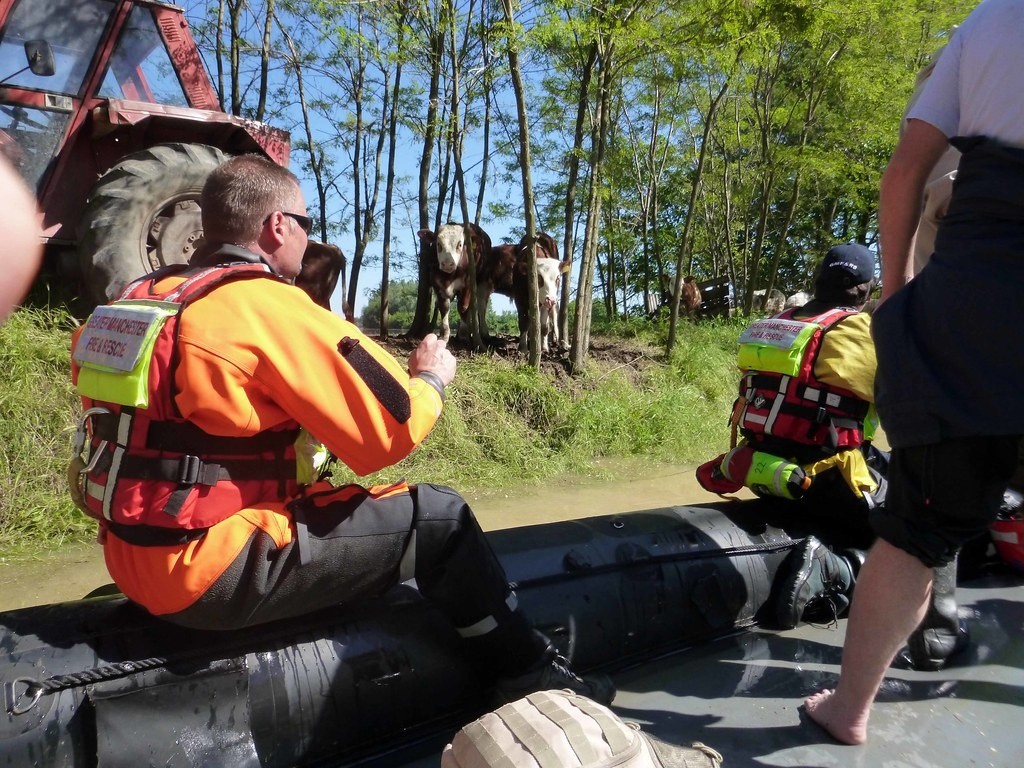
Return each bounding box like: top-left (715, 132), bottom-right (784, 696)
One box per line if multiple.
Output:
top-left (1, 1), bottom-right (295, 305)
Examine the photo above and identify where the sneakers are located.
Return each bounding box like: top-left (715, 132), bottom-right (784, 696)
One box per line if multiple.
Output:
top-left (496, 656), bottom-right (619, 708)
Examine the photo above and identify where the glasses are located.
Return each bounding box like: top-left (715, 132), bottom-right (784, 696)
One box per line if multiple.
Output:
top-left (262, 211), bottom-right (314, 236)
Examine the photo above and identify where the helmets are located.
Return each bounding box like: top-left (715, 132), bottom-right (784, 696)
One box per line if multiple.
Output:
top-left (816, 242), bottom-right (875, 289)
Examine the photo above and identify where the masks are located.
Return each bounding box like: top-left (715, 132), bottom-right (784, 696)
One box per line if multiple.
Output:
top-left (855, 285), bottom-right (871, 305)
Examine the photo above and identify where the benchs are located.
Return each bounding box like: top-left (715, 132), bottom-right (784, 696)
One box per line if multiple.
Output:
top-left (659, 274), bottom-right (733, 324)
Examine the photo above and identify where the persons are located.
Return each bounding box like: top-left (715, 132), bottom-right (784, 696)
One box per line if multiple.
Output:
top-left (72, 155), bottom-right (617, 703)
top-left (798, 0), bottom-right (1024, 743)
top-left (0, 155), bottom-right (42, 325)
top-left (737, 242), bottom-right (901, 630)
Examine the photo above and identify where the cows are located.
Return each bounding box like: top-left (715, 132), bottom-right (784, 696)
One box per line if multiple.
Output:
top-left (416, 217), bottom-right (567, 362)
top-left (658, 267), bottom-right (817, 316)
top-left (301, 238), bottom-right (352, 317)
